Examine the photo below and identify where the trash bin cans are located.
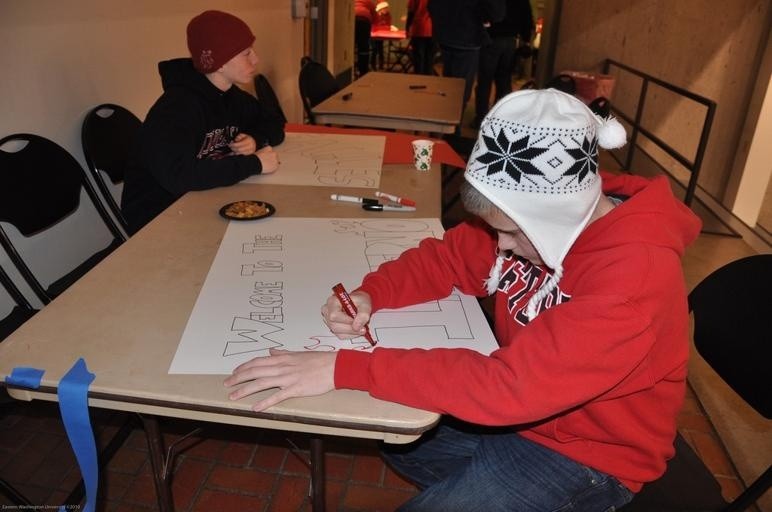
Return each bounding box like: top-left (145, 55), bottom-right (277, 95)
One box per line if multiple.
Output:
top-left (560, 70), bottom-right (615, 108)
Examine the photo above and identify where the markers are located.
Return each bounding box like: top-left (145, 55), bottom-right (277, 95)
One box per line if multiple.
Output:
top-left (343, 92), bottom-right (353, 100)
top-left (409, 85), bottom-right (426, 89)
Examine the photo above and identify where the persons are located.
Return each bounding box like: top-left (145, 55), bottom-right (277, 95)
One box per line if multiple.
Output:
top-left (464, 1), bottom-right (536, 128)
top-left (119, 10), bottom-right (287, 234)
top-left (353, 1), bottom-right (436, 79)
top-left (214, 87), bottom-right (703, 510)
top-left (426, 1), bottom-right (480, 114)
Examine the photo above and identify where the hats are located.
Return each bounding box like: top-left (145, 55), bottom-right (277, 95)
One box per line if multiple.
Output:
top-left (184, 8), bottom-right (257, 75)
top-left (462, 86), bottom-right (605, 270)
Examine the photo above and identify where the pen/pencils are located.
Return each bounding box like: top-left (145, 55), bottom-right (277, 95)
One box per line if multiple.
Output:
top-left (331, 191), bottom-right (416, 211)
top-left (415, 90), bottom-right (446, 96)
top-left (332, 283), bottom-right (375, 347)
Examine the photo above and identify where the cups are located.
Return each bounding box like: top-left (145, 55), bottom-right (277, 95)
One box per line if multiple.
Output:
top-left (411, 138), bottom-right (435, 173)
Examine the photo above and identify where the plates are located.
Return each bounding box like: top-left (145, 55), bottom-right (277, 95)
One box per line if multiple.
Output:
top-left (219, 199), bottom-right (277, 222)
